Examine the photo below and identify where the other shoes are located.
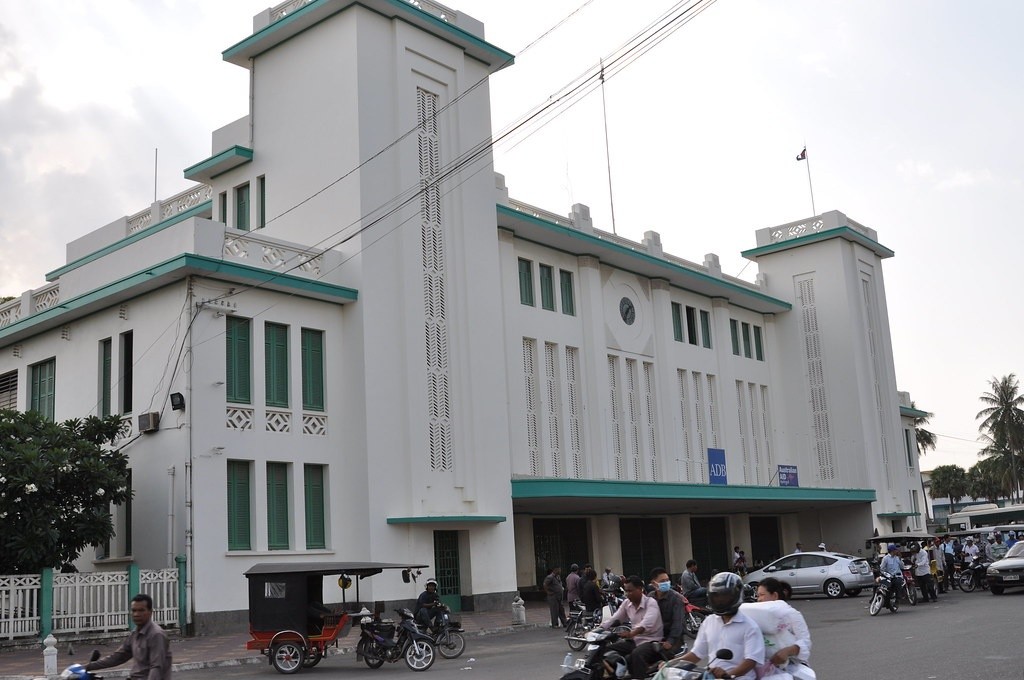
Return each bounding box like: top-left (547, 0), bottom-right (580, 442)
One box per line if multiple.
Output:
top-left (923, 598), bottom-right (929, 602)
top-left (430, 626), bottom-right (439, 632)
top-left (933, 598), bottom-right (938, 602)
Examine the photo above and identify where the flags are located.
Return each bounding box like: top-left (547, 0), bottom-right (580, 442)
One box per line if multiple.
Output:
top-left (797, 150), bottom-right (806, 160)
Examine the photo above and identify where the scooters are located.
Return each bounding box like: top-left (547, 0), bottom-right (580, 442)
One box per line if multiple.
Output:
top-left (645, 640), bottom-right (736, 680)
top-left (60, 642), bottom-right (133, 680)
top-left (557, 614), bottom-right (688, 680)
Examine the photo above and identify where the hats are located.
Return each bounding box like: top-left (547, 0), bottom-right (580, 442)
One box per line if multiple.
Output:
top-left (796, 542), bottom-right (802, 545)
top-left (570, 564), bottom-right (578, 570)
top-left (987, 535), bottom-right (994, 539)
top-left (611, 575), bottom-right (622, 582)
top-left (932, 537), bottom-right (941, 543)
top-left (965, 536), bottom-right (973, 540)
top-left (1007, 531), bottom-right (1015, 536)
top-left (818, 543), bottom-right (825, 548)
top-left (995, 531), bottom-right (1001, 536)
top-left (620, 575), bottom-right (626, 584)
top-left (1018, 532), bottom-right (1024, 536)
top-left (888, 545), bottom-right (899, 552)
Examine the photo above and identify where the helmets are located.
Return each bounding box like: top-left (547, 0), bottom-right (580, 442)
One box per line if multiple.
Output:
top-left (426, 578), bottom-right (438, 592)
top-left (706, 572), bottom-right (743, 616)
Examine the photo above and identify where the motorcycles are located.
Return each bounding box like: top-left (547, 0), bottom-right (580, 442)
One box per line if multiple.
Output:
top-left (354, 607), bottom-right (436, 672)
top-left (394, 601), bottom-right (467, 660)
top-left (867, 560), bottom-right (901, 617)
top-left (958, 552), bottom-right (994, 592)
top-left (564, 576), bottom-right (759, 651)
top-left (898, 561), bottom-right (919, 607)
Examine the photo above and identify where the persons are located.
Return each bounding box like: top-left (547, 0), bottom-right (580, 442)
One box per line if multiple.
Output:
top-left (880, 522), bottom-right (1024, 603)
top-left (414, 580), bottom-right (441, 634)
top-left (543, 542), bottom-right (827, 680)
top-left (83, 595), bottom-right (171, 680)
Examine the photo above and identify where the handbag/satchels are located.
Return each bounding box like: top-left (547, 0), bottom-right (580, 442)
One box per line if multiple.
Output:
top-left (755, 660), bottom-right (816, 680)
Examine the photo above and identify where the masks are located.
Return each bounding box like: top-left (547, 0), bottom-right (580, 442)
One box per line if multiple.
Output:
top-left (652, 581), bottom-right (670, 592)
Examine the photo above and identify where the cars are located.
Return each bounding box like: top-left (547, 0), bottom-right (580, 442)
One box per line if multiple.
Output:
top-left (986, 541), bottom-right (1024, 595)
top-left (738, 551), bottom-right (874, 599)
